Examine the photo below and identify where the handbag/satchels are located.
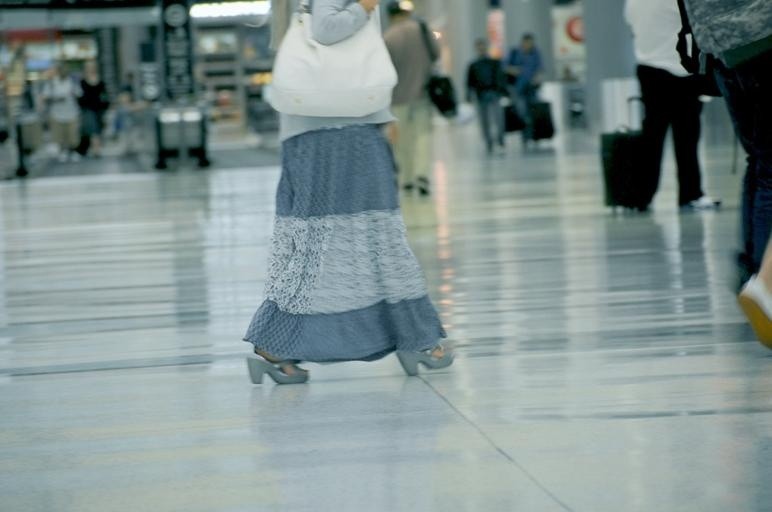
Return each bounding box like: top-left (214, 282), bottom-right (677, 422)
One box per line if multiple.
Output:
top-left (678, 1), bottom-right (722, 102)
top-left (264, 0), bottom-right (398, 117)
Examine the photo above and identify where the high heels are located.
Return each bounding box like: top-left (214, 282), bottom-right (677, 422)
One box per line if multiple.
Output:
top-left (247, 355), bottom-right (307, 384)
top-left (398, 347), bottom-right (452, 376)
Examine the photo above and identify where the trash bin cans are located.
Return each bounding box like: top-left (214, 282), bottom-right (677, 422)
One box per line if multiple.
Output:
top-left (154, 108), bottom-right (211, 169)
top-left (0, 122), bottom-right (32, 183)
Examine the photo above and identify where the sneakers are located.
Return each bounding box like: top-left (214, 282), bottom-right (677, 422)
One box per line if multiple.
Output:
top-left (680, 196), bottom-right (720, 209)
top-left (734, 256), bottom-right (771, 350)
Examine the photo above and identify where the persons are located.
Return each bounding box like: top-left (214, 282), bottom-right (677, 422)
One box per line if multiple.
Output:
top-left (241, 0), bottom-right (455, 384)
top-left (40, 59), bottom-right (111, 165)
top-left (684, 0), bottom-right (772, 351)
top-left (625, 0), bottom-right (722, 209)
top-left (382, 1), bottom-right (441, 196)
top-left (464, 33), bottom-right (547, 154)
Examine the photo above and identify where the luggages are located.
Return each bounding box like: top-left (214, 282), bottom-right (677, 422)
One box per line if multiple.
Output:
top-left (531, 103), bottom-right (553, 140)
top-left (602, 96), bottom-right (642, 212)
